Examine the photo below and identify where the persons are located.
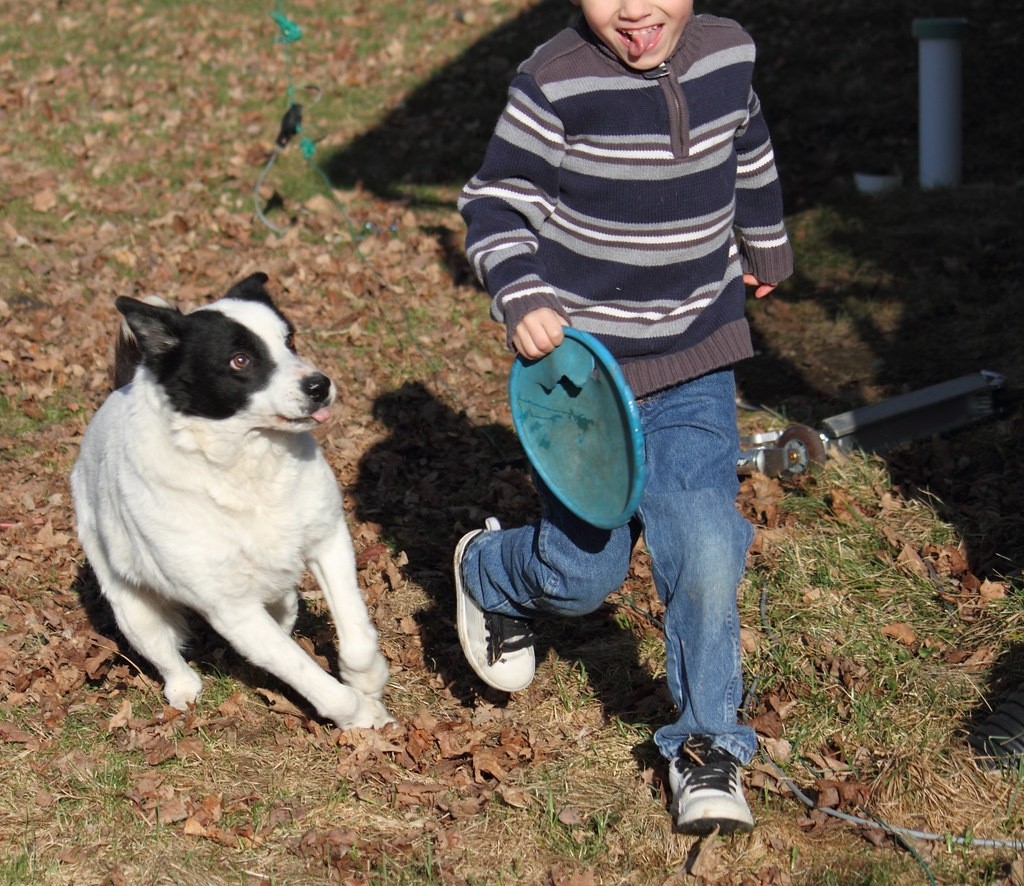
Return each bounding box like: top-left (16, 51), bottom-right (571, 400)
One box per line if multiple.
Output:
top-left (450, 0), bottom-right (798, 827)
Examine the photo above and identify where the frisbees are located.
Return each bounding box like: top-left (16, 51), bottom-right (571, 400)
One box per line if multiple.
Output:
top-left (502, 321), bottom-right (659, 531)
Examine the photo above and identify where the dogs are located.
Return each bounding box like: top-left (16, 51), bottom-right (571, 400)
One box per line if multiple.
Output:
top-left (67, 271), bottom-right (393, 733)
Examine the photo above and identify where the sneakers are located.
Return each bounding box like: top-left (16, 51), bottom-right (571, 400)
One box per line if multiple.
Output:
top-left (669, 735), bottom-right (755, 835)
top-left (454, 517), bottom-right (536, 692)
